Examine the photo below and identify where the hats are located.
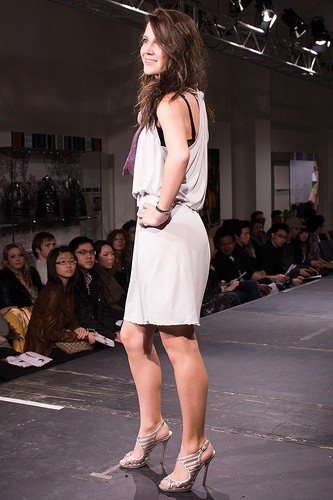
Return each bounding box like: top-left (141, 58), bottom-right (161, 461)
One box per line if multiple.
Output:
top-left (267, 222), bottom-right (290, 237)
top-left (284, 216), bottom-right (306, 228)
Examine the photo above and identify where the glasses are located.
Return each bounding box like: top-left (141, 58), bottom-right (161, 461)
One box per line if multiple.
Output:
top-left (320, 224), bottom-right (325, 227)
top-left (55, 260), bottom-right (77, 264)
top-left (74, 249), bottom-right (96, 256)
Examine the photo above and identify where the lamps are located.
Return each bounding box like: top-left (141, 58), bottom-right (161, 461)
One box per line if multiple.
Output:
top-left (257, 0), bottom-right (274, 21)
top-left (310, 16), bottom-right (328, 46)
top-left (239, 0), bottom-right (253, 12)
top-left (281, 8), bottom-right (309, 37)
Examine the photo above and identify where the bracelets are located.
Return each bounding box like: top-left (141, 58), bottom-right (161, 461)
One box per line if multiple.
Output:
top-left (156, 201), bottom-right (176, 213)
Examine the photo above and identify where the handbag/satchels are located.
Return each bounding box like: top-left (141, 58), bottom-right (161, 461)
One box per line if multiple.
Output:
top-left (55, 329), bottom-right (95, 353)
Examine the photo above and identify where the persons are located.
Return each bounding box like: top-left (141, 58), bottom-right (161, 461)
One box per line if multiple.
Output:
top-left (0, 219), bottom-right (138, 358)
top-left (200, 200), bottom-right (333, 317)
top-left (311, 165), bottom-right (318, 189)
top-left (119, 8), bottom-right (215, 492)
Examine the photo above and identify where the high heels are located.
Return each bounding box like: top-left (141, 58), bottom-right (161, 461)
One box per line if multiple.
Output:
top-left (118, 419), bottom-right (172, 468)
top-left (158, 439), bottom-right (216, 492)
